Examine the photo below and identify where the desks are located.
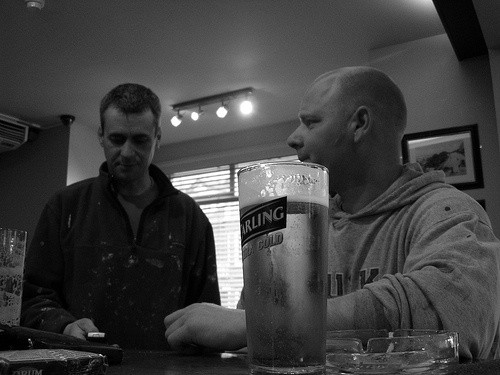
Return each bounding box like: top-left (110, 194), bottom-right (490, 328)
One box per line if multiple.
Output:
top-left (104, 349), bottom-right (500, 375)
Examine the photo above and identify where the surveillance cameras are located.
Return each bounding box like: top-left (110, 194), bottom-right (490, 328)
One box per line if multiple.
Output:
top-left (60, 115), bottom-right (76, 124)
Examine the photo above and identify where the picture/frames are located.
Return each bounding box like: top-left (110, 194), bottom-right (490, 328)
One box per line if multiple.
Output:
top-left (400, 123), bottom-right (484, 191)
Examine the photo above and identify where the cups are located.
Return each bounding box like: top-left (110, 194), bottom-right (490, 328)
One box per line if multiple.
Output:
top-left (0, 228), bottom-right (27, 326)
top-left (237, 161), bottom-right (329, 375)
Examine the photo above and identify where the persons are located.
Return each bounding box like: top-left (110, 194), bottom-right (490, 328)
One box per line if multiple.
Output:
top-left (164, 66), bottom-right (500, 361)
top-left (20, 83), bottom-right (223, 356)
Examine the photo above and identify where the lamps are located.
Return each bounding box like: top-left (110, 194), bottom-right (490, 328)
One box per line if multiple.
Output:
top-left (171, 87), bottom-right (254, 127)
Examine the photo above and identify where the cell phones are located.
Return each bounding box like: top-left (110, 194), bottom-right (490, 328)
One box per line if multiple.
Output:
top-left (85, 332), bottom-right (110, 344)
